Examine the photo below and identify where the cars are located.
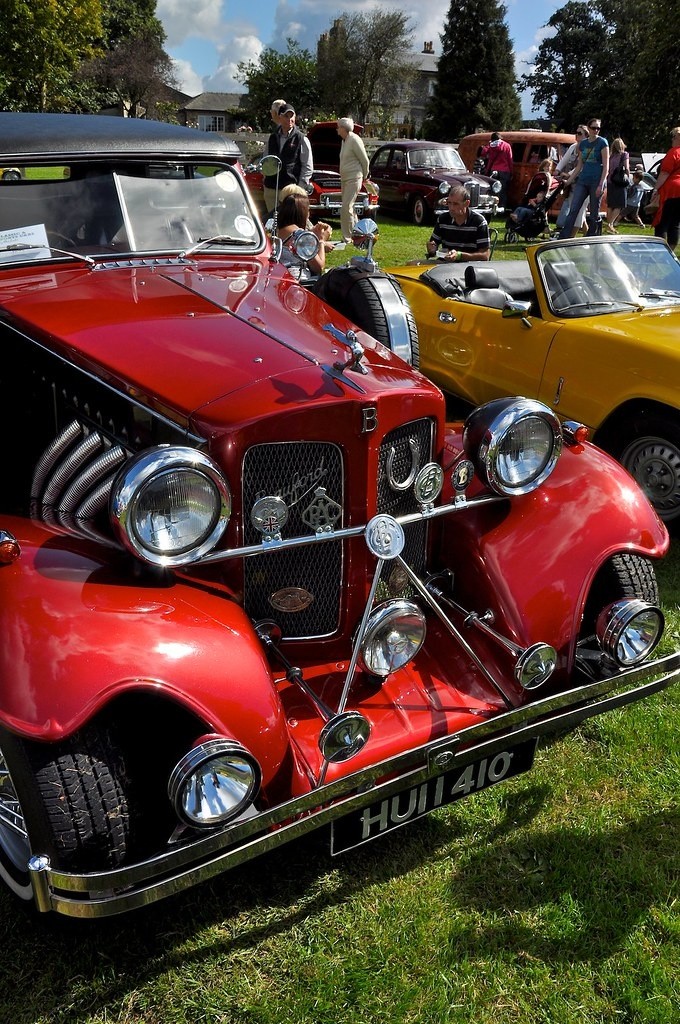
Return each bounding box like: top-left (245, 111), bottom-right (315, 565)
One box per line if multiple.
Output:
top-left (241, 121), bottom-right (380, 222)
top-left (0, 107), bottom-right (680, 924)
top-left (367, 138), bottom-right (505, 225)
top-left (624, 152), bottom-right (669, 224)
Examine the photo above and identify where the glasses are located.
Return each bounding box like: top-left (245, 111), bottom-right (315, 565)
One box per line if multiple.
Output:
top-left (576, 131), bottom-right (586, 136)
top-left (588, 126), bottom-right (601, 130)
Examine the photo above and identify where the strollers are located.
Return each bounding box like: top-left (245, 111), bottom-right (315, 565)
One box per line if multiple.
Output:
top-left (503, 170), bottom-right (567, 245)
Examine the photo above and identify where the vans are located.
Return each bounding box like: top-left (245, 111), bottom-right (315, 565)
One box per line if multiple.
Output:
top-left (456, 127), bottom-right (610, 220)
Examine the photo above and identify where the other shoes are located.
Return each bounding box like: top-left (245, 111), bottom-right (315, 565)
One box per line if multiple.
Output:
top-left (640, 224), bottom-right (645, 228)
top-left (510, 213), bottom-right (517, 221)
top-left (613, 222), bottom-right (619, 227)
top-left (606, 225), bottom-right (619, 234)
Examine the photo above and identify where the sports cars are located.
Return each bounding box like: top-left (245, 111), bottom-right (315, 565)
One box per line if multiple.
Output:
top-left (380, 231), bottom-right (680, 535)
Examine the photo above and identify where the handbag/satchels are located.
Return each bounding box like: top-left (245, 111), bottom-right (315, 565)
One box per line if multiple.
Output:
top-left (610, 151), bottom-right (630, 188)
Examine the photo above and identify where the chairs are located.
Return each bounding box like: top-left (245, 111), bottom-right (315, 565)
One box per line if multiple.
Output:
top-left (465, 266), bottom-right (515, 310)
top-left (544, 261), bottom-right (596, 310)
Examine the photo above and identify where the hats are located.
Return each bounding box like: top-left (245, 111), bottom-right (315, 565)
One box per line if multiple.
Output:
top-left (278, 104), bottom-right (295, 116)
top-left (630, 170), bottom-right (644, 180)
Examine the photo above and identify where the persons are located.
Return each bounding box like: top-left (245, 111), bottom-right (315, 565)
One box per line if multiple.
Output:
top-left (472, 132), bottom-right (512, 203)
top-left (260, 100), bottom-right (332, 284)
top-left (338, 115), bottom-right (370, 241)
top-left (605, 136), bottom-right (630, 233)
top-left (511, 188), bottom-right (546, 224)
top-left (651, 125), bottom-right (680, 251)
top-left (428, 184), bottom-right (491, 264)
top-left (537, 120), bottom-right (656, 240)
